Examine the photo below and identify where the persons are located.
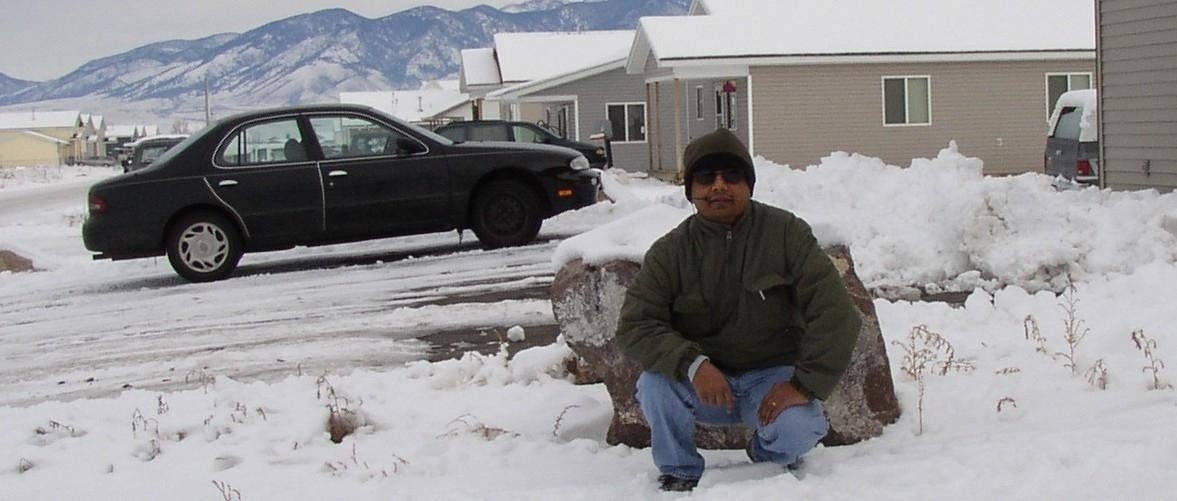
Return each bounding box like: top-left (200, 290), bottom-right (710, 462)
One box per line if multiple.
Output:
top-left (617, 126), bottom-right (861, 492)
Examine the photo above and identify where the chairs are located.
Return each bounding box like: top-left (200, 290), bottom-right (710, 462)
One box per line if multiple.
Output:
top-left (283, 139), bottom-right (306, 161)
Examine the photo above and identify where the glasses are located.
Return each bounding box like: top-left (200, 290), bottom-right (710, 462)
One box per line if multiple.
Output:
top-left (693, 169), bottom-right (744, 184)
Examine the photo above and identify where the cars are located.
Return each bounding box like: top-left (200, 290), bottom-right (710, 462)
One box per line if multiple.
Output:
top-left (432, 118), bottom-right (609, 171)
top-left (81, 102), bottom-right (606, 285)
top-left (119, 134), bottom-right (191, 174)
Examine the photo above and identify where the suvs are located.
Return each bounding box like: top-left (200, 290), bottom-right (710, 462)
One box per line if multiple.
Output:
top-left (1039, 84), bottom-right (1103, 192)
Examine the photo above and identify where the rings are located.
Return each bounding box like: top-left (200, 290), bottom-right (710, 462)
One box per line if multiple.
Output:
top-left (772, 403), bottom-right (776, 408)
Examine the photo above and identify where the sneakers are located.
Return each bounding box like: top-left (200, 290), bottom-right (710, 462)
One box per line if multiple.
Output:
top-left (659, 475), bottom-right (698, 491)
top-left (746, 439), bottom-right (763, 462)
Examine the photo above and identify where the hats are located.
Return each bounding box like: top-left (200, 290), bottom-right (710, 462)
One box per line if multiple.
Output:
top-left (683, 128), bottom-right (755, 203)
